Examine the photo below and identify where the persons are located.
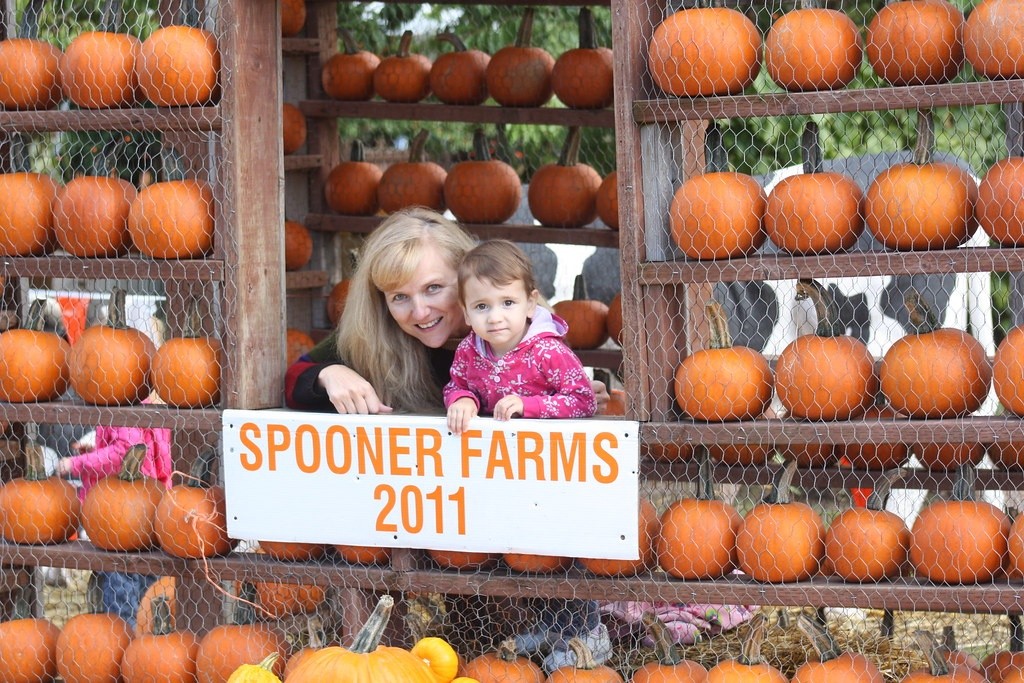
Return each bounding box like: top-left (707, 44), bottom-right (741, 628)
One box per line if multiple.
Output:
top-left (443, 239), bottom-right (596, 434)
top-left (56, 391), bottom-right (173, 508)
top-left (283, 206), bottom-right (611, 417)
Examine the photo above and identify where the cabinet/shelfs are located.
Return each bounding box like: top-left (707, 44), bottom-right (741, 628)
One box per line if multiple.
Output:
top-left (0, 0), bottom-right (1024, 683)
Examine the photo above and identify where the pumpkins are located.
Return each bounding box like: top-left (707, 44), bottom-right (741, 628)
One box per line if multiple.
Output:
top-left (0, 0), bottom-right (1024, 683)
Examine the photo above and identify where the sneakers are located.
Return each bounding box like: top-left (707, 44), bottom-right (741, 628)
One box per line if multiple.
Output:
top-left (543, 624), bottom-right (614, 673)
top-left (513, 624), bottom-right (552, 655)
